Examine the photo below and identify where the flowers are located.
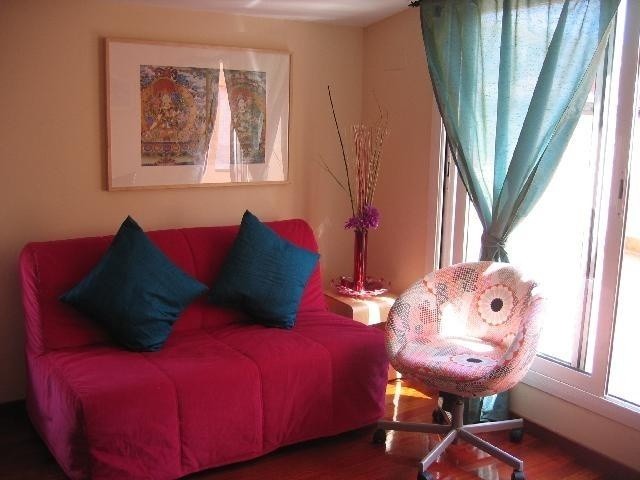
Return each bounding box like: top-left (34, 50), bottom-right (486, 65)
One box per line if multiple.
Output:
top-left (342, 200), bottom-right (382, 234)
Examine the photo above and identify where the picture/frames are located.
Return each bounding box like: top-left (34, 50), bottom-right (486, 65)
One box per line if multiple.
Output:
top-left (100, 34), bottom-right (294, 193)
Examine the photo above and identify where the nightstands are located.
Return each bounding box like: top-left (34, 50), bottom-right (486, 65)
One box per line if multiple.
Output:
top-left (323, 290), bottom-right (398, 328)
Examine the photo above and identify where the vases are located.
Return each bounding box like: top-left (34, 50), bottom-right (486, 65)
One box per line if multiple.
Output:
top-left (351, 228), bottom-right (370, 293)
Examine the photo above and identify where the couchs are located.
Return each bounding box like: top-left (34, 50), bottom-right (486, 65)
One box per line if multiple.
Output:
top-left (20, 218), bottom-right (389, 480)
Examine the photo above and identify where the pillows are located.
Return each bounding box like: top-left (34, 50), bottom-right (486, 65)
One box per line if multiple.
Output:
top-left (59, 210), bottom-right (321, 349)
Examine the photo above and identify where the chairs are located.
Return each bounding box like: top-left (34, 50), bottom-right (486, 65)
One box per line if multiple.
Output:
top-left (371, 260), bottom-right (542, 480)
